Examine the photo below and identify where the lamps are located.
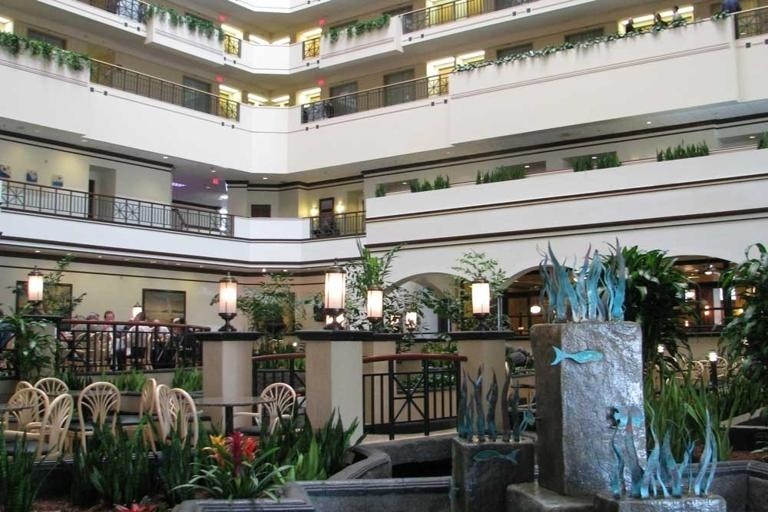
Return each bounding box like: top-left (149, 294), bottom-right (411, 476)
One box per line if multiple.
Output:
top-left (323, 259), bottom-right (383, 332)
top-left (471, 270), bottom-right (491, 331)
top-left (217, 272), bottom-right (238, 332)
top-left (26, 265), bottom-right (45, 315)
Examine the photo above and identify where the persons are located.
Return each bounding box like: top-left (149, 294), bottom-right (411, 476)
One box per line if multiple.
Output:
top-left (720, 0), bottom-right (741, 13)
top-left (671, 5), bottom-right (683, 21)
top-left (625, 19), bottom-right (634, 33)
top-left (69, 310), bottom-right (191, 370)
top-left (0, 310), bottom-right (16, 370)
top-left (652, 12), bottom-right (661, 23)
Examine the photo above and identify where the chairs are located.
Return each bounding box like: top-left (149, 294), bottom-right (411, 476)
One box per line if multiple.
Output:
top-left (0, 376), bottom-right (298, 465)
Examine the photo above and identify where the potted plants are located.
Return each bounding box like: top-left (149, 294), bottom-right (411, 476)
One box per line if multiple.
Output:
top-left (717, 240), bottom-right (768, 451)
